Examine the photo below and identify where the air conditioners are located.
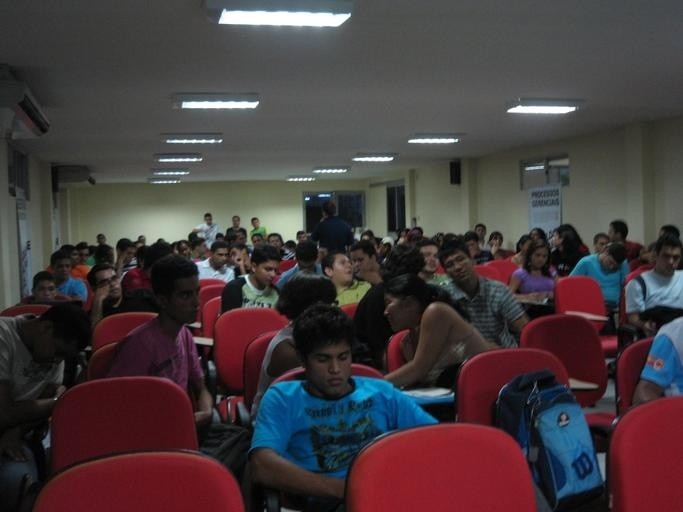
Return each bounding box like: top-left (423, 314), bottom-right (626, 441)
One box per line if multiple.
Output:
top-left (0, 78), bottom-right (51, 138)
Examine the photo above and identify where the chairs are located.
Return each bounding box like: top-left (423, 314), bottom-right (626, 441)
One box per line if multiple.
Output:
top-left (0, 251), bottom-right (683, 512)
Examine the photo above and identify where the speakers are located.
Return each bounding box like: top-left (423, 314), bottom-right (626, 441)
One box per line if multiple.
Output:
top-left (449, 160), bottom-right (461, 185)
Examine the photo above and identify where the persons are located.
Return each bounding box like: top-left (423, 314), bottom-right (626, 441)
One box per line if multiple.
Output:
top-left (383, 274), bottom-right (500, 425)
top-left (252, 268), bottom-right (338, 417)
top-left (52, 251), bottom-right (89, 301)
top-left (625, 234), bottom-right (682, 337)
top-left (207, 244), bottom-right (284, 377)
top-left (353, 244), bottom-right (466, 376)
top-left (87, 264), bottom-right (161, 333)
top-left (1, 302), bottom-right (93, 480)
top-left (462, 219), bottom-right (657, 334)
top-left (247, 304), bottom-right (438, 512)
top-left (267, 202), bottom-right (459, 306)
top-left (170, 212), bottom-right (268, 283)
top-left (31, 271), bottom-right (57, 304)
top-left (634, 317), bottom-right (681, 407)
top-left (438, 240), bottom-right (530, 349)
top-left (105, 254), bottom-right (250, 481)
top-left (47, 234), bottom-right (171, 288)
top-left (660, 225), bottom-right (680, 239)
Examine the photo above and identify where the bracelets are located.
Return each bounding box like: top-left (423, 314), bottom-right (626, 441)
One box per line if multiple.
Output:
top-left (52, 396), bottom-right (58, 401)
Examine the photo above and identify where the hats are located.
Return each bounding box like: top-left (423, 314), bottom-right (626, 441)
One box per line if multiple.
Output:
top-left (607, 239), bottom-right (629, 264)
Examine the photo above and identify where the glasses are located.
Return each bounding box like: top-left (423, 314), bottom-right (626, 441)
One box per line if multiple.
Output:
top-left (95, 274), bottom-right (119, 288)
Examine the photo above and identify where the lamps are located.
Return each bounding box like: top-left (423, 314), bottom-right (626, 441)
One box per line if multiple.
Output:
top-left (409, 133), bottom-right (461, 147)
top-left (506, 101), bottom-right (577, 118)
top-left (212, 6), bottom-right (354, 31)
top-left (150, 132), bottom-right (225, 187)
top-left (170, 90), bottom-right (262, 112)
top-left (354, 152), bottom-right (397, 165)
top-left (286, 165), bottom-right (349, 183)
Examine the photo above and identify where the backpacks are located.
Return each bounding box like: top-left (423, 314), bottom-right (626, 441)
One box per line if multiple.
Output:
top-left (494, 368), bottom-right (609, 510)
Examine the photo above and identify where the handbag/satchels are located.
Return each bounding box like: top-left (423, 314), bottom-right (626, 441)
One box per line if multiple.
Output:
top-left (637, 306), bottom-right (682, 339)
